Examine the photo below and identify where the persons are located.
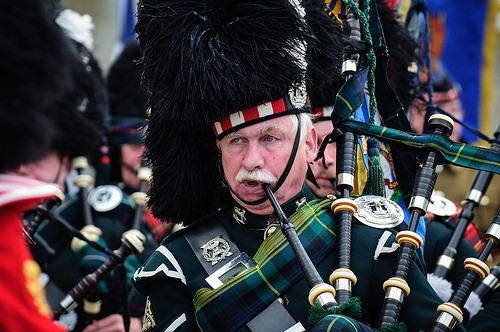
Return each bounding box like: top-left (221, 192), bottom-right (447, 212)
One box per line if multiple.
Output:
top-left (0, 86), bottom-right (500, 332)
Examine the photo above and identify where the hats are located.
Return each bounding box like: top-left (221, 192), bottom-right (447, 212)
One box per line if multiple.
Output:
top-left (133, 0), bottom-right (310, 226)
top-left (301, 0), bottom-right (369, 121)
top-left (107, 43), bottom-right (150, 144)
top-left (417, 76), bottom-right (465, 100)
top-left (0, 0), bottom-right (92, 166)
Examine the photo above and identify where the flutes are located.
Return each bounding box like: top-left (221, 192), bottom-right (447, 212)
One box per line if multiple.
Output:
top-left (23, 157), bottom-right (189, 322)
top-left (263, 0), bottom-right (500, 332)
